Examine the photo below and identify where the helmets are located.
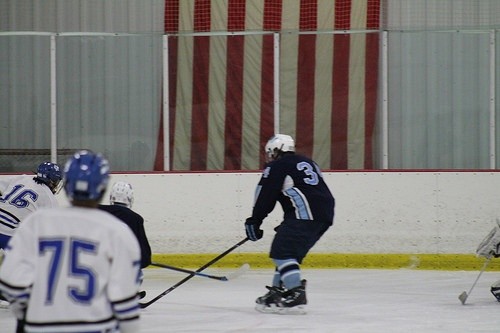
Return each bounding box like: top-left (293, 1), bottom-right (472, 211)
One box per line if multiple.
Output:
top-left (110, 180), bottom-right (134, 207)
top-left (38, 162), bottom-right (63, 193)
top-left (265, 133), bottom-right (295, 156)
top-left (64, 151), bottom-right (110, 199)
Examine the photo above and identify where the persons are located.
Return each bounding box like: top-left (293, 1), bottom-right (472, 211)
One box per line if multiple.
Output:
top-left (244, 135), bottom-right (335, 314)
top-left (99, 181), bottom-right (151, 299)
top-left (477, 219), bottom-right (500, 302)
top-left (0, 148), bottom-right (142, 333)
top-left (0, 161), bottom-right (64, 309)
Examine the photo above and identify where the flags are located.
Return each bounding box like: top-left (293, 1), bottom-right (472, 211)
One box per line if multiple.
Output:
top-left (153, 0), bottom-right (381, 172)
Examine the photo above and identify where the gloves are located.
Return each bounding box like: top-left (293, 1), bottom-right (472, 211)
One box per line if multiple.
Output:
top-left (245, 217), bottom-right (263, 240)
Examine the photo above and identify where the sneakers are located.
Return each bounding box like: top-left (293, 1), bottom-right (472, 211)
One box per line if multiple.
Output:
top-left (267, 280), bottom-right (306, 313)
top-left (256, 286), bottom-right (284, 310)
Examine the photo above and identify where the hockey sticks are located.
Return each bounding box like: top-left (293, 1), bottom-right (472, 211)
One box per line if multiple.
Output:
top-left (459, 249), bottom-right (494, 306)
top-left (149, 261), bottom-right (250, 282)
top-left (135, 237), bottom-right (250, 310)
